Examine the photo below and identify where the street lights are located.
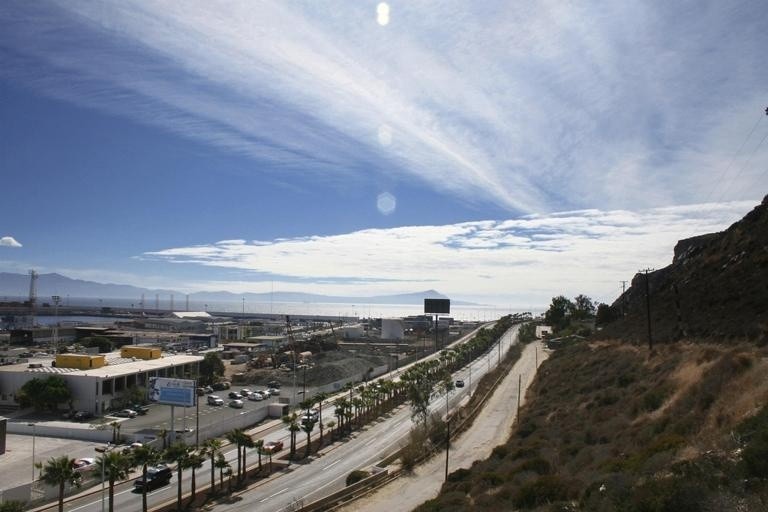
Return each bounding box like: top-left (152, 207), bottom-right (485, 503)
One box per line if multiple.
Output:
top-left (51, 295), bottom-right (60, 355)
top-left (28, 424), bottom-right (35, 482)
top-left (95, 446), bottom-right (104, 512)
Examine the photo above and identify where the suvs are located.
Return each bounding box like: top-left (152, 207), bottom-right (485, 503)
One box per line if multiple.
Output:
top-left (134, 466), bottom-right (172, 491)
top-left (301, 411), bottom-right (319, 424)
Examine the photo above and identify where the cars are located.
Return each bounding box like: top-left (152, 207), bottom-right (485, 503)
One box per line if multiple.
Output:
top-left (131, 405), bottom-right (149, 414)
top-left (456, 381), bottom-right (464, 387)
top-left (196, 385), bottom-right (214, 395)
top-left (261, 442), bottom-right (283, 454)
top-left (75, 411), bottom-right (88, 419)
top-left (230, 392), bottom-right (243, 399)
top-left (230, 400), bottom-right (244, 408)
top-left (549, 335), bottom-right (585, 349)
top-left (20, 353), bottom-right (34, 358)
top-left (207, 396), bottom-right (224, 406)
top-left (113, 409), bottom-right (137, 417)
top-left (241, 387), bottom-right (280, 401)
